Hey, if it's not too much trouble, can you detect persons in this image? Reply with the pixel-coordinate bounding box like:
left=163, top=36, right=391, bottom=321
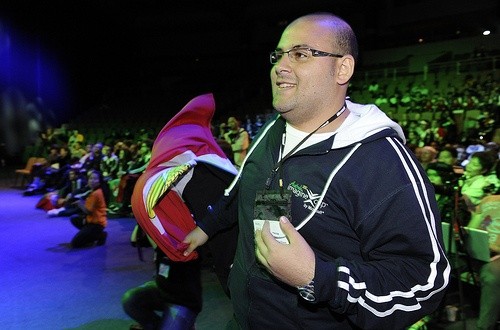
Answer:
left=23, top=79, right=500, bottom=330
left=177, top=14, right=448, bottom=330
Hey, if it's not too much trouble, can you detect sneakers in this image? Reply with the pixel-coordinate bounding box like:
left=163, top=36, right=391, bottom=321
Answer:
left=109, top=207, right=125, bottom=215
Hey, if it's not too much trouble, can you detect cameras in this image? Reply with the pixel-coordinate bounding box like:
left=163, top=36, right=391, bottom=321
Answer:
left=222, top=125, right=231, bottom=132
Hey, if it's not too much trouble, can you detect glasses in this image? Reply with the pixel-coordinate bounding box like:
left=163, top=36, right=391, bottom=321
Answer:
left=270, top=45, right=343, bottom=64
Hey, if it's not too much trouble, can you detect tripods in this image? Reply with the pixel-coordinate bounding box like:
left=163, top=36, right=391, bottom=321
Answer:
left=440, top=186, right=482, bottom=330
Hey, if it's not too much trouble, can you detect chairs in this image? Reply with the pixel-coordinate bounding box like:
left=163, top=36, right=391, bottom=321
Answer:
left=442, top=222, right=500, bottom=288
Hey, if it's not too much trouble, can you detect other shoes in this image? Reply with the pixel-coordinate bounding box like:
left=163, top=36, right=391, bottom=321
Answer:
left=16, top=170, right=30, bottom=174
left=96, top=232, right=108, bottom=246
